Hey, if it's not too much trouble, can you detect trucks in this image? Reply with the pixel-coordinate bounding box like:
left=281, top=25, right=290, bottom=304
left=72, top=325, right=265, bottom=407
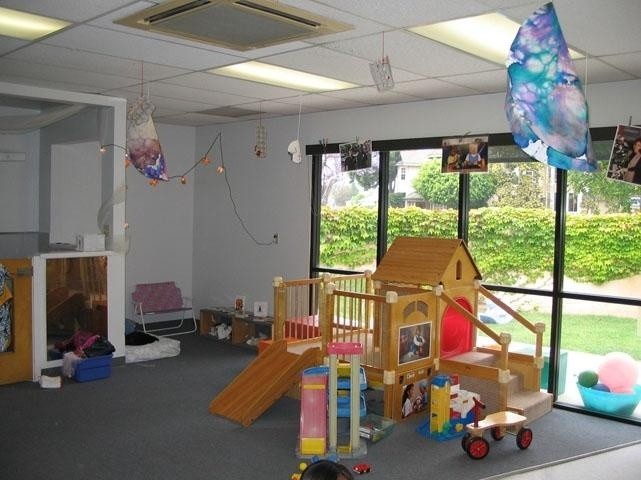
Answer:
left=630, top=197, right=641, bottom=213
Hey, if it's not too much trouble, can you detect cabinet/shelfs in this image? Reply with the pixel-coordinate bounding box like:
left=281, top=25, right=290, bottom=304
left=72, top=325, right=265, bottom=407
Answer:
left=200, top=307, right=274, bottom=352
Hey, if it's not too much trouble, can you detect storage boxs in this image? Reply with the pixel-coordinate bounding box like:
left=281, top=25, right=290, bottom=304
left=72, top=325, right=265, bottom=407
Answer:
left=60, top=351, right=113, bottom=382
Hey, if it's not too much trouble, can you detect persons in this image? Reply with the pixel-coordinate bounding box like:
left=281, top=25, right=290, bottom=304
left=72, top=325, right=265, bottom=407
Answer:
left=446, top=146, right=463, bottom=171
left=345, top=148, right=358, bottom=170
left=619, top=138, right=641, bottom=186
left=416, top=382, right=430, bottom=408
left=466, top=142, right=486, bottom=170
left=356, top=144, right=369, bottom=169
left=408, top=324, right=428, bottom=357
left=401, top=383, right=416, bottom=418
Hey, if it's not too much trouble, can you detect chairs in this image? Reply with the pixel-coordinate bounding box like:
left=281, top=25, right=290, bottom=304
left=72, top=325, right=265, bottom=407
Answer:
left=130, top=281, right=198, bottom=337
